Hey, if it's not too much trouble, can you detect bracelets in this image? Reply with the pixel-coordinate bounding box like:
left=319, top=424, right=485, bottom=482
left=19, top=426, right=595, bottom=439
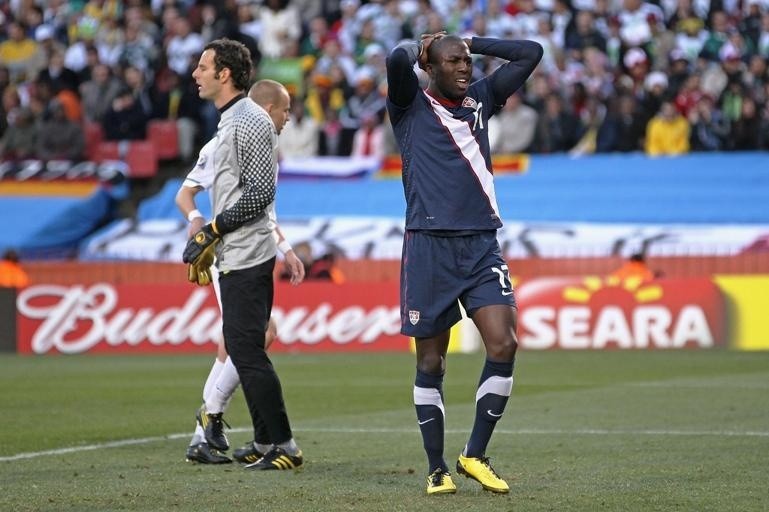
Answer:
left=416, top=41, right=425, bottom=59
left=278, top=240, right=292, bottom=255
left=186, top=209, right=203, bottom=222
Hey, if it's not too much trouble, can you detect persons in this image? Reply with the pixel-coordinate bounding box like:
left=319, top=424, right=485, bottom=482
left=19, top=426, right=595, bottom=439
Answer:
left=299, top=1, right=769, bottom=160
left=183, top=39, right=305, bottom=473
left=175, top=77, right=307, bottom=467
left=2, top=1, right=303, bottom=162
left=0, top=248, right=30, bottom=289
left=380, top=30, right=543, bottom=495
left=609, top=237, right=658, bottom=282
left=304, top=239, right=343, bottom=284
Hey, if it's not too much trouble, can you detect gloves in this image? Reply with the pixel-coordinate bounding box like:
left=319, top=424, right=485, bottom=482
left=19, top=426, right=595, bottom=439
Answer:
left=182, top=224, right=220, bottom=268
left=186, top=262, right=212, bottom=285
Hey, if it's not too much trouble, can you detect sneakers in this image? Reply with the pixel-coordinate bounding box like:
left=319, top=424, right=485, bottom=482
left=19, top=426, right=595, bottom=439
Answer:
left=233, top=440, right=263, bottom=463
left=185, top=441, right=233, bottom=465
left=195, top=403, right=232, bottom=450
left=245, top=444, right=305, bottom=470
left=456, top=453, right=509, bottom=494
left=426, top=467, right=456, bottom=495
